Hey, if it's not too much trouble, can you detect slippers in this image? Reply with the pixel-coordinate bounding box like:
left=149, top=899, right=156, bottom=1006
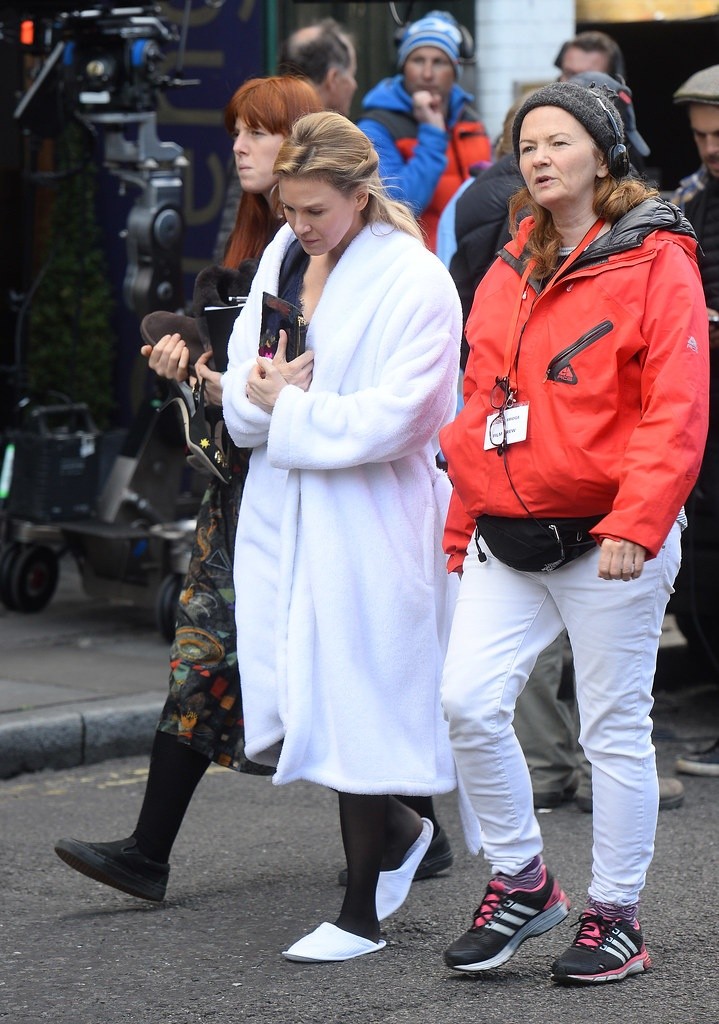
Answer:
left=374, top=817, right=434, bottom=921
left=282, top=921, right=387, bottom=961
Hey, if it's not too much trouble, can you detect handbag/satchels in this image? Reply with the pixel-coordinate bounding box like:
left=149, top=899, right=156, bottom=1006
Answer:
left=475, top=514, right=607, bottom=572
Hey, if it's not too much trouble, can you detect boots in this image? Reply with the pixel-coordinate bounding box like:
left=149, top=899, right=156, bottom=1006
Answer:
left=141, top=259, right=260, bottom=380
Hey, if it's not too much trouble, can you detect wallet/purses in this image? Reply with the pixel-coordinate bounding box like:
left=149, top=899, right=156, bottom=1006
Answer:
left=258, top=291, right=309, bottom=362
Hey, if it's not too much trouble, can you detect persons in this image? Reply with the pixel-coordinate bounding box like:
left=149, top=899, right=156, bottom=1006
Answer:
left=277, top=11, right=719, bottom=813
left=439, top=83, right=709, bottom=983
left=53, top=76, right=454, bottom=899
left=220, top=112, right=464, bottom=964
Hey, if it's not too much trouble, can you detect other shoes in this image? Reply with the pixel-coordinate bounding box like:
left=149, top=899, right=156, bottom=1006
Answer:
left=676, top=740, right=719, bottom=776
left=532, top=776, right=579, bottom=807
left=576, top=777, right=684, bottom=811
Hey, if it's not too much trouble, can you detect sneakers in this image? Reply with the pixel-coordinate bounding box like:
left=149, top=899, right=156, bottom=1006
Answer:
left=339, top=826, right=452, bottom=886
left=56, top=838, right=170, bottom=902
left=550, top=910, right=652, bottom=984
left=444, top=864, right=571, bottom=971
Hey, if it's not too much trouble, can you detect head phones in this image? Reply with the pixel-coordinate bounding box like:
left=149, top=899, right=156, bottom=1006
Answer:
left=590, top=89, right=630, bottom=179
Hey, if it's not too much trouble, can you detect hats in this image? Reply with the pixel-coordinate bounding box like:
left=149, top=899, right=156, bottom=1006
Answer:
left=674, top=64, right=719, bottom=104
left=569, top=71, right=651, bottom=156
left=511, top=81, right=630, bottom=179
left=397, top=10, right=463, bottom=83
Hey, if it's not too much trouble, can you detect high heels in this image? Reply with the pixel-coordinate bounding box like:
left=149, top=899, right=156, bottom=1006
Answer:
left=156, top=377, right=233, bottom=485
